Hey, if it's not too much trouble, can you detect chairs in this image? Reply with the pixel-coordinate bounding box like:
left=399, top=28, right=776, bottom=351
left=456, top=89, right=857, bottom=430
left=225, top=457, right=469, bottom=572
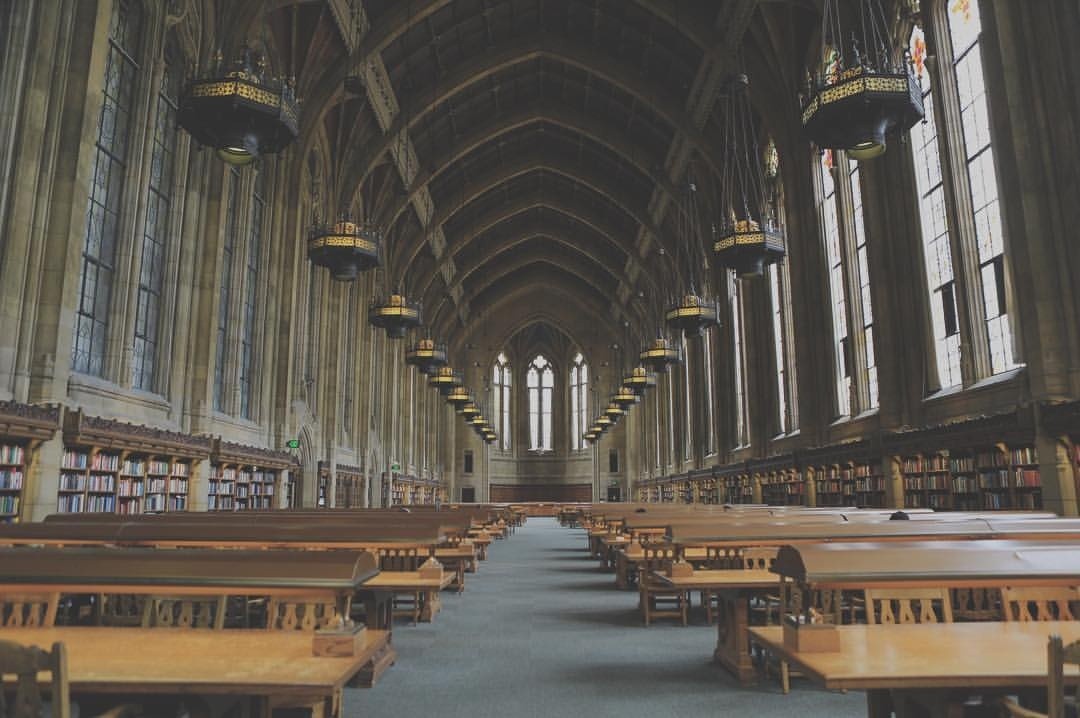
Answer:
left=1, top=508, right=1080, bottom=718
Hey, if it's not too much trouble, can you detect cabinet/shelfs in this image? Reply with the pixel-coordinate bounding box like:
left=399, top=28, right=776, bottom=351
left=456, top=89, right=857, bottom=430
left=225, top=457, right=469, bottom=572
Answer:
left=2, top=397, right=1079, bottom=520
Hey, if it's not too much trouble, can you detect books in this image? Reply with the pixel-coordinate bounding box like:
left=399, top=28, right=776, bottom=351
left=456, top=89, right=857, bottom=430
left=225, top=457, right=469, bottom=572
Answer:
left=318, top=473, right=372, bottom=509
left=639, top=479, right=718, bottom=506
left=393, top=484, right=450, bottom=506
left=722, top=478, right=752, bottom=504
left=903, top=444, right=1042, bottom=511
left=55, top=443, right=190, bottom=514
left=0, top=443, right=27, bottom=524
left=813, top=461, right=886, bottom=508
left=761, top=470, right=804, bottom=505
left=208, top=464, right=276, bottom=511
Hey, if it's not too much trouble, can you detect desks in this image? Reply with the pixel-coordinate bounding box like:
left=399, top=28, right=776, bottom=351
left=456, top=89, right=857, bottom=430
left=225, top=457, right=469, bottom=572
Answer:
left=362, top=570, right=457, bottom=622
left=746, top=621, right=1080, bottom=718
left=469, top=523, right=509, bottom=537
left=448, top=536, right=490, bottom=560
left=599, top=536, right=654, bottom=572
left=613, top=550, right=741, bottom=589
left=466, top=529, right=503, bottom=544
left=588, top=530, right=620, bottom=559
left=0, top=623, right=389, bottom=718
left=379, top=547, right=478, bottom=592
left=639, top=565, right=791, bottom=625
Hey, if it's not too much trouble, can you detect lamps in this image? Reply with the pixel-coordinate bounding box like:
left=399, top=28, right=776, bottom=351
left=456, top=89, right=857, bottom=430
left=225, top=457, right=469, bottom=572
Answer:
left=176, top=1, right=923, bottom=445
